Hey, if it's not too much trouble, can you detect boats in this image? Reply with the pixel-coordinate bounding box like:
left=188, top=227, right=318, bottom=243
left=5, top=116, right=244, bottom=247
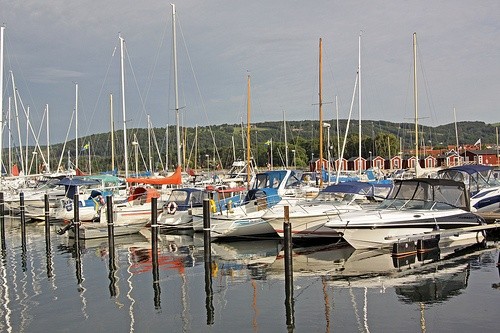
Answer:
left=323, top=176, right=488, bottom=250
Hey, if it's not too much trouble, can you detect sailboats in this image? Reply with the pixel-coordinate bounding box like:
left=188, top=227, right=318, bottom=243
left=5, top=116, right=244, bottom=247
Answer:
left=0, top=2, right=500, bottom=240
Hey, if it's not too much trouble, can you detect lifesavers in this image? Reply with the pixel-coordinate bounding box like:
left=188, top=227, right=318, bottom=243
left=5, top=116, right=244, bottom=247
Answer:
left=167, top=201, right=177, bottom=214
left=210, top=200, right=217, bottom=213
